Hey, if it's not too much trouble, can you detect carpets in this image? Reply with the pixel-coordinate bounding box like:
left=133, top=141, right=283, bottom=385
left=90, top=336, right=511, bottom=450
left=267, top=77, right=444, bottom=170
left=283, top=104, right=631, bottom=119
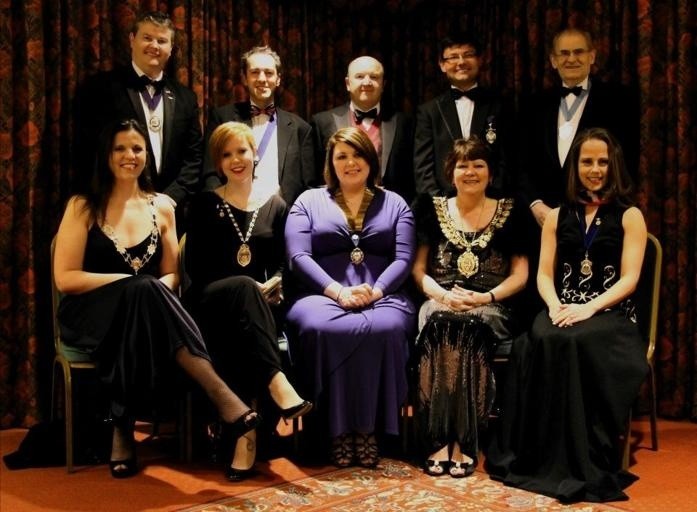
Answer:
left=174, top=457, right=634, bottom=512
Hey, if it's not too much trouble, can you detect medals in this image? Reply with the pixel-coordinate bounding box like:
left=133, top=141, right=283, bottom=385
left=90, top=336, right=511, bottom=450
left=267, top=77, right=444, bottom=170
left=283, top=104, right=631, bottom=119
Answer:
left=579, top=260, right=593, bottom=276
left=457, top=249, right=479, bottom=279
left=351, top=247, right=363, bottom=266
left=237, top=244, right=252, bottom=266
left=149, top=113, right=161, bottom=132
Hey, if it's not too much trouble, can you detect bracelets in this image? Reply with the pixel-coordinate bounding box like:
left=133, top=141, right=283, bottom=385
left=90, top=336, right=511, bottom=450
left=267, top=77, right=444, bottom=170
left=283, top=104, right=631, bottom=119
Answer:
left=336, top=287, right=344, bottom=302
left=488, top=289, right=495, bottom=303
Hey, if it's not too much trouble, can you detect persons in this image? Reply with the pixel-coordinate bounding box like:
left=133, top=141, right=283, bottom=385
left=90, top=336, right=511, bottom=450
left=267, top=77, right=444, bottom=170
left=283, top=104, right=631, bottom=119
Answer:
left=202, top=45, right=316, bottom=219
left=70, top=13, right=203, bottom=205
left=412, top=133, right=531, bottom=477
left=284, top=127, right=416, bottom=469
left=185, top=121, right=314, bottom=479
left=54, top=118, right=265, bottom=479
left=517, top=27, right=641, bottom=230
left=311, top=56, right=417, bottom=206
left=483, top=126, right=650, bottom=503
left=413, top=34, right=518, bottom=199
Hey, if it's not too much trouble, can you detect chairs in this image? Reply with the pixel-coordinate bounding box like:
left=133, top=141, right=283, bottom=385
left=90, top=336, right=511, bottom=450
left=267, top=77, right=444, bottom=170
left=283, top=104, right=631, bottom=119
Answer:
left=488, top=325, right=514, bottom=365
left=49, top=231, right=192, bottom=475
left=622, top=230, right=664, bottom=471
left=282, top=330, right=407, bottom=458
left=177, top=231, right=190, bottom=297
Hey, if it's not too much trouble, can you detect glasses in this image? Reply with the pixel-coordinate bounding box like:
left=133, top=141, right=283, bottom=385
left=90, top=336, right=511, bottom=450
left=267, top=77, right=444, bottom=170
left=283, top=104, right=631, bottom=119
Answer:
left=443, top=51, right=474, bottom=60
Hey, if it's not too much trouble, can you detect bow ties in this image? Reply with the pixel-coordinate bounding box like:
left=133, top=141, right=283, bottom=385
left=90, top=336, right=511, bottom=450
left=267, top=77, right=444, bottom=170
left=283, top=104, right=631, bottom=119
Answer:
left=250, top=106, right=276, bottom=117
left=450, top=87, right=478, bottom=100
left=138, top=75, right=164, bottom=90
left=353, top=109, right=377, bottom=121
left=560, top=87, right=582, bottom=97
